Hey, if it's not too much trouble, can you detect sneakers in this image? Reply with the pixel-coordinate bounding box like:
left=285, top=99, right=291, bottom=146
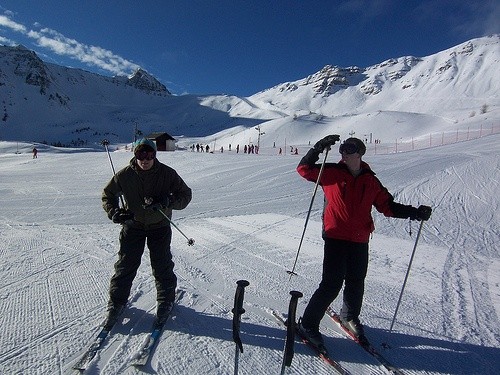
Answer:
left=158, top=195, right=172, bottom=209
left=340, top=309, right=364, bottom=338
left=107, top=304, right=123, bottom=320
left=296, top=323, right=324, bottom=349
left=157, top=302, right=173, bottom=318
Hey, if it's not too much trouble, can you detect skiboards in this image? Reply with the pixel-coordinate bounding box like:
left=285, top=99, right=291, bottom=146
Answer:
left=291, top=154, right=299, bottom=155
left=271, top=306, right=403, bottom=375
left=73, top=289, right=183, bottom=370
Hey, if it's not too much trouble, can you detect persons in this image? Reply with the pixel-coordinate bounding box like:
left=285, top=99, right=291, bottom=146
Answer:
left=244, top=144, right=259, bottom=155
left=229, top=144, right=231, bottom=151
left=298, top=134, right=431, bottom=351
left=374, top=139, right=381, bottom=144
left=190, top=143, right=210, bottom=153
left=279, top=148, right=282, bottom=156
left=125, top=144, right=128, bottom=150
left=220, top=147, right=223, bottom=153
left=33, top=147, right=37, bottom=159
left=290, top=145, right=293, bottom=152
left=308, top=141, right=310, bottom=145
left=295, top=148, right=298, bottom=155
left=365, top=139, right=367, bottom=143
left=102, top=138, right=191, bottom=333
left=236, top=145, right=239, bottom=154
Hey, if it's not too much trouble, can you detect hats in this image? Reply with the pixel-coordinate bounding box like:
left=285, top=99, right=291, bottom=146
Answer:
left=133, top=137, right=156, bottom=155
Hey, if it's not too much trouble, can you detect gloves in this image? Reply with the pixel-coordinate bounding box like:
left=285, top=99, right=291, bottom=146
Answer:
left=314, top=135, right=340, bottom=153
left=112, top=208, right=134, bottom=223
left=410, top=205, right=432, bottom=221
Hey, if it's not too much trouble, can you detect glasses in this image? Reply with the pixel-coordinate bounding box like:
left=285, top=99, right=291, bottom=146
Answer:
left=135, top=150, right=155, bottom=161
left=339, top=144, right=358, bottom=154
